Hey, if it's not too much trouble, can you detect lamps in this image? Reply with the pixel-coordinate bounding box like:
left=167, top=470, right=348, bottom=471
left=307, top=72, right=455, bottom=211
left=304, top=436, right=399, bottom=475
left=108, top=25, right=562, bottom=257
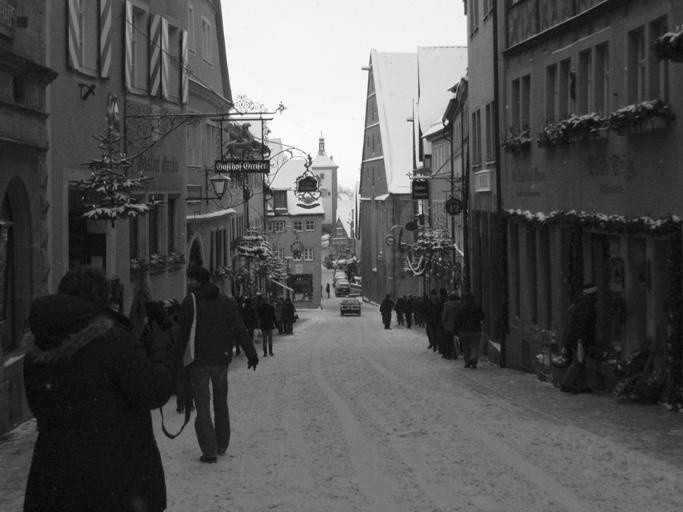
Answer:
left=185, top=167, right=231, bottom=203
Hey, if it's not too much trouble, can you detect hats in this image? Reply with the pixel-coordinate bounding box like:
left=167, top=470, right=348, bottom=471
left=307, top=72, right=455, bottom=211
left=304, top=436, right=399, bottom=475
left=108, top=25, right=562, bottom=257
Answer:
left=187, top=265, right=210, bottom=283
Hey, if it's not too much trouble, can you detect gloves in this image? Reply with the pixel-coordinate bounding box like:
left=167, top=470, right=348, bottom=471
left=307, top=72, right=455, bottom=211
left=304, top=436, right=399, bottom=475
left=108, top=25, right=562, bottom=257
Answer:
left=247, top=353, right=259, bottom=371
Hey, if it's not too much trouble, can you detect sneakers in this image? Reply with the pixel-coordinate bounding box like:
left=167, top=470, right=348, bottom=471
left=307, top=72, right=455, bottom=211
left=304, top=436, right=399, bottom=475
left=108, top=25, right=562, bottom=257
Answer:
left=200, top=454, right=217, bottom=463
left=428, top=344, right=478, bottom=369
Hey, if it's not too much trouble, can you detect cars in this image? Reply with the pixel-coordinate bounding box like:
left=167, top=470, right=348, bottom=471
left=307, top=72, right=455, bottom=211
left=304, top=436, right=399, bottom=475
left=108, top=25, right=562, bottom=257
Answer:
left=332, top=267, right=362, bottom=318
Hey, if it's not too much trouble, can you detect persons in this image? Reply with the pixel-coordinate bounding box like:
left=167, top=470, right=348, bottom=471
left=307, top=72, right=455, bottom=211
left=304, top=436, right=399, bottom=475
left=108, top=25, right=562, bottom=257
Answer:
left=378, top=287, right=486, bottom=371
left=171, top=264, right=260, bottom=464
left=22, top=271, right=173, bottom=512
left=564, top=274, right=597, bottom=361
left=231, top=291, right=297, bottom=359
left=325, top=282, right=331, bottom=299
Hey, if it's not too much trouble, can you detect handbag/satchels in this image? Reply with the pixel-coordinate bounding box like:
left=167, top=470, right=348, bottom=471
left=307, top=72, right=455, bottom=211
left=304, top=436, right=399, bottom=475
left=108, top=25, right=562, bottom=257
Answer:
left=182, top=327, right=196, bottom=368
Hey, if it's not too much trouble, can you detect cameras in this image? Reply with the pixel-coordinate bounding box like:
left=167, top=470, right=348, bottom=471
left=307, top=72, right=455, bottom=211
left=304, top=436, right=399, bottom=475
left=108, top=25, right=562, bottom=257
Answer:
left=145, top=298, right=181, bottom=326
